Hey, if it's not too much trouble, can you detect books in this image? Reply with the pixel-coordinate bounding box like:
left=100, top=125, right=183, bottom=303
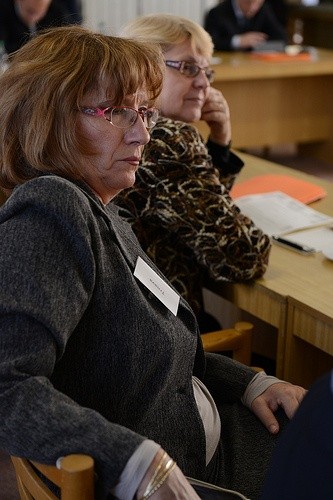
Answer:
left=227, top=173, right=333, bottom=259
left=246, top=37, right=312, bottom=65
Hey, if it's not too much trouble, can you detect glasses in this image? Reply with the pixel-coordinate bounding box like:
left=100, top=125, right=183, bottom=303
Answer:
left=55, top=104, right=161, bottom=129
left=164, top=59, right=215, bottom=82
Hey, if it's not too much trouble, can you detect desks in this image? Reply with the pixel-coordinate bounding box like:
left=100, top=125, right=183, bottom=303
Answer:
left=283, top=272, right=333, bottom=389
left=188, top=45, right=332, bottom=172
left=200, top=147, right=333, bottom=381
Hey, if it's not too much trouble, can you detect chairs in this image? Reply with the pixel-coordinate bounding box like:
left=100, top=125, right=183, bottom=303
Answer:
left=9, top=453, right=95, bottom=500
left=194, top=320, right=254, bottom=368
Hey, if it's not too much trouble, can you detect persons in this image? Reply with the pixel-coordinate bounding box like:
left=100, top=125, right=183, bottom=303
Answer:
left=0, top=25, right=308, bottom=500
left=0, top=0, right=81, bottom=65
left=205, top=0, right=289, bottom=53
left=117, top=13, right=275, bottom=361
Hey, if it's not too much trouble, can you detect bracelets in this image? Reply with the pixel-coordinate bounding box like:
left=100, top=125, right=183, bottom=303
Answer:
left=139, top=451, right=177, bottom=500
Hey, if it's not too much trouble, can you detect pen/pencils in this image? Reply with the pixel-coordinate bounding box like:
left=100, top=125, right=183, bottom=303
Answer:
left=272, top=234, right=310, bottom=253
